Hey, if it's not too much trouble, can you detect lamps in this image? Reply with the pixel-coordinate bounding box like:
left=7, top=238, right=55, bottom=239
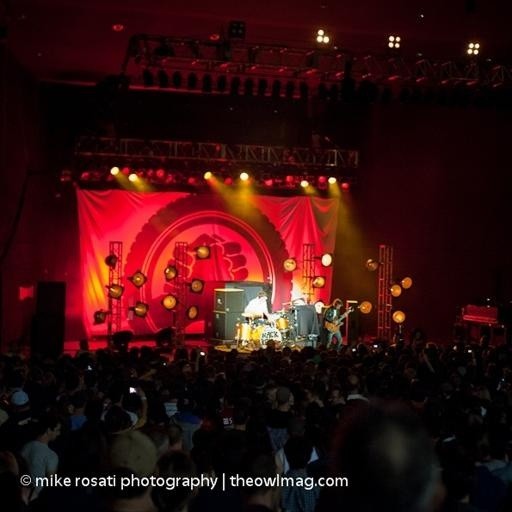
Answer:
left=94, top=245, right=415, bottom=324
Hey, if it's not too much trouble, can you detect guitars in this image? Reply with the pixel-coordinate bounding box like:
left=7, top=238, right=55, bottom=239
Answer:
left=324, top=306, right=354, bottom=334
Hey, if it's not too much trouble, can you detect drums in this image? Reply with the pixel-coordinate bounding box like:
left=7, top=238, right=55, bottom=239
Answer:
left=252, top=325, right=282, bottom=350
left=254, top=318, right=271, bottom=327
left=275, top=316, right=290, bottom=333
left=234, top=324, right=241, bottom=341
left=240, top=323, right=252, bottom=341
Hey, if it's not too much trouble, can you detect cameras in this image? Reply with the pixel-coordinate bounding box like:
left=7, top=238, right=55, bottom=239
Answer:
left=372, top=344, right=379, bottom=349
left=467, top=349, right=473, bottom=354
left=199, top=351, right=206, bottom=356
left=351, top=347, right=357, bottom=353
left=129, top=386, right=137, bottom=394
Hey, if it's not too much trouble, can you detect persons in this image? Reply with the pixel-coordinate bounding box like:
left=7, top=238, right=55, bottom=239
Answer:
left=2, top=322, right=511, bottom=512
left=240, top=289, right=270, bottom=323
left=320, top=297, right=348, bottom=351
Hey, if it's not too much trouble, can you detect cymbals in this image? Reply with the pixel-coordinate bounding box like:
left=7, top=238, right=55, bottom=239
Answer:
left=274, top=310, right=289, bottom=313
left=241, top=312, right=262, bottom=319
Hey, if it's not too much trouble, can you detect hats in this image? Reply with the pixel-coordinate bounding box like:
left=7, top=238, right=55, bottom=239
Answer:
left=106, top=429, right=160, bottom=480
left=10, top=391, right=30, bottom=406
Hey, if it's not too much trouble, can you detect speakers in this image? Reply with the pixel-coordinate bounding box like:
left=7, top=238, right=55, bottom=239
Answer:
left=112, top=330, right=135, bottom=352
left=213, top=311, right=241, bottom=340
left=297, top=305, right=319, bottom=336
left=213, top=288, right=245, bottom=314
left=32, top=280, right=66, bottom=359
left=155, top=326, right=177, bottom=346
